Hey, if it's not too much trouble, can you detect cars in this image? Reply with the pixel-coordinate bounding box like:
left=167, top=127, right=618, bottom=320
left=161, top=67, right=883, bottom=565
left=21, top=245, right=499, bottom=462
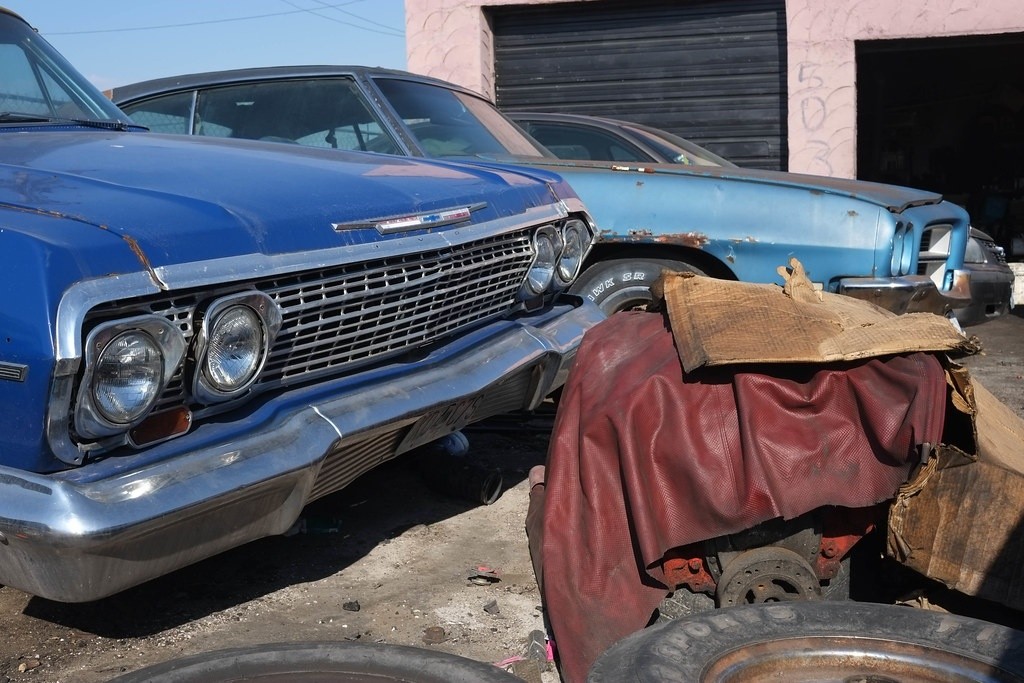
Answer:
left=953, top=226, right=1015, bottom=328
left=49, top=64, right=969, bottom=346
left=351, top=111, right=744, bottom=171
left=0, top=7, right=611, bottom=605
